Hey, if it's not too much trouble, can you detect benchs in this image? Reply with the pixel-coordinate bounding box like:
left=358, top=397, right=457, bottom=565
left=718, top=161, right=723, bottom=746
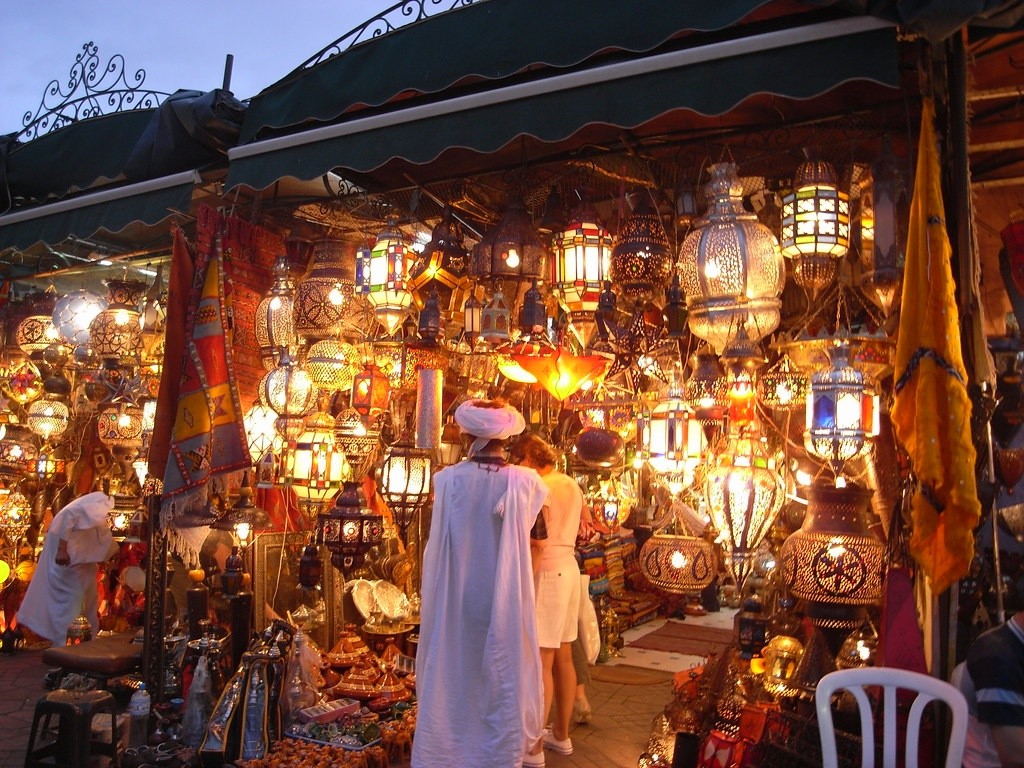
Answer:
left=39, top=628, right=144, bottom=741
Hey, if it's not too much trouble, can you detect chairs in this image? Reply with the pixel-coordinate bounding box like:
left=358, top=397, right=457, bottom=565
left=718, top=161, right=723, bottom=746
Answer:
left=816, top=667, right=969, bottom=768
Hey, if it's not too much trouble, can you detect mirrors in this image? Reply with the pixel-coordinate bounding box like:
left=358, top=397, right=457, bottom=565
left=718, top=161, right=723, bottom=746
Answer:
left=145, top=494, right=252, bottom=743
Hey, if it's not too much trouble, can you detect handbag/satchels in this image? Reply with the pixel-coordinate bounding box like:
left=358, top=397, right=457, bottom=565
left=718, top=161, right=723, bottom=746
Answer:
left=577, top=574, right=600, bottom=665
left=868, top=566, right=930, bottom=705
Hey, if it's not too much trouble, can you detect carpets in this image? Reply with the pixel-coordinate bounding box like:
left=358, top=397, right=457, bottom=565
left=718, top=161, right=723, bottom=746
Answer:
left=623, top=619, right=732, bottom=657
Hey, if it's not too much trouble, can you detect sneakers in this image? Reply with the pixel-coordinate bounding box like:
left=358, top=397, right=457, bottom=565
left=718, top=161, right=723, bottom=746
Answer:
left=524, top=751, right=546, bottom=768
left=543, top=725, right=573, bottom=756
left=574, top=699, right=592, bottom=723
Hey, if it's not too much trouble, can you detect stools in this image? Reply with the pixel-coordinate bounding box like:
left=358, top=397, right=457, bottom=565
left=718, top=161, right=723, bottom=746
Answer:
left=24, top=690, right=118, bottom=768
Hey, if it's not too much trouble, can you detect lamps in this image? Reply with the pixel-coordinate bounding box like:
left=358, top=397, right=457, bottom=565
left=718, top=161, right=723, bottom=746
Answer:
left=0, top=147, right=891, bottom=698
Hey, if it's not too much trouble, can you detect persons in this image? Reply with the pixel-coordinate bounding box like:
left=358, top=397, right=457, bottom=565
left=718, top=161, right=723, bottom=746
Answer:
left=975, top=373, right=1023, bottom=560
left=407, top=397, right=594, bottom=768
left=958, top=572, right=1024, bottom=768
left=16, top=490, right=114, bottom=649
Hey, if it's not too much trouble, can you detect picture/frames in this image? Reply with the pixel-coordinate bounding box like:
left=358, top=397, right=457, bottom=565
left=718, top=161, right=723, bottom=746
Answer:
left=253, top=531, right=343, bottom=652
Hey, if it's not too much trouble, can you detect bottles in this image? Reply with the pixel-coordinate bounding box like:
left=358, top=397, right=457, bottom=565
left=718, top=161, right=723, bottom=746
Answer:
left=767, top=598, right=800, bottom=641
left=740, top=601, right=767, bottom=659
left=128, top=683, right=150, bottom=747
